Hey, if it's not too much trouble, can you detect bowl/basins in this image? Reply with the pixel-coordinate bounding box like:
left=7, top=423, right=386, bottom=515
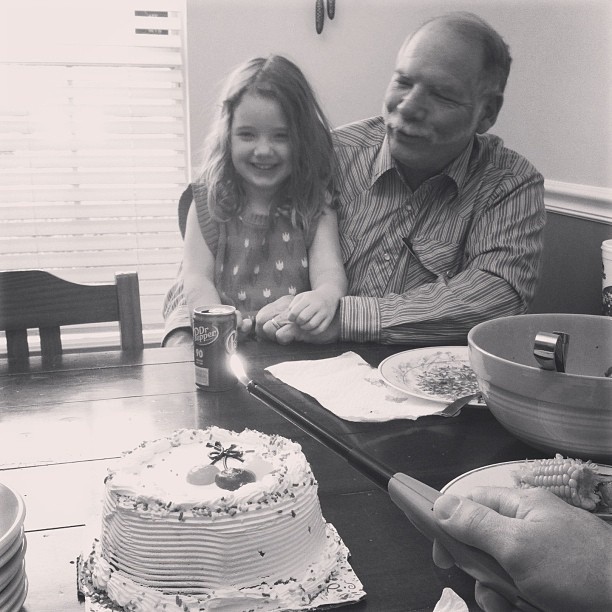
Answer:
left=467, top=312, right=612, bottom=462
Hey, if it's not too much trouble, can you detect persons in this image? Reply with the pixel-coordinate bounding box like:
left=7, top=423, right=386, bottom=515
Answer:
left=432, top=485, right=612, bottom=611
left=161, top=11, right=547, bottom=348
left=178, top=55, right=349, bottom=344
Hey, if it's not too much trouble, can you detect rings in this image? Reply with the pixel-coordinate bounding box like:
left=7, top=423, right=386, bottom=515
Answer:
left=271, top=318, right=281, bottom=329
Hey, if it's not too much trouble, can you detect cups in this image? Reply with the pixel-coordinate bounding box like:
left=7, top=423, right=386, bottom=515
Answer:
left=600, top=238, right=612, bottom=317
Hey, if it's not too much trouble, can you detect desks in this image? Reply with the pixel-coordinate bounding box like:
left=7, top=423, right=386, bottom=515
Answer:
left=1, top=345, right=547, bottom=611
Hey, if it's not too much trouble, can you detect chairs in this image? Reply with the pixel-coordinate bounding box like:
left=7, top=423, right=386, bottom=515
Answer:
left=178, top=183, right=193, bottom=239
left=0, top=270, right=143, bottom=355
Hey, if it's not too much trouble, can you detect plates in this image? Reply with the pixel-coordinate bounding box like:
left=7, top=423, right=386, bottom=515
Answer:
left=0, top=574, right=25, bottom=612
left=438, top=458, right=612, bottom=525
left=0, top=558, right=26, bottom=607
left=0, top=526, right=20, bottom=557
left=0, top=568, right=21, bottom=593
left=377, top=343, right=487, bottom=408
left=0, top=533, right=28, bottom=586
left=0, top=480, right=26, bottom=550
left=10, top=583, right=29, bottom=612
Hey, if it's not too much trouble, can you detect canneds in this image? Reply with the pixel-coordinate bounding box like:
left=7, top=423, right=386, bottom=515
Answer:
left=192, top=304, right=239, bottom=390
